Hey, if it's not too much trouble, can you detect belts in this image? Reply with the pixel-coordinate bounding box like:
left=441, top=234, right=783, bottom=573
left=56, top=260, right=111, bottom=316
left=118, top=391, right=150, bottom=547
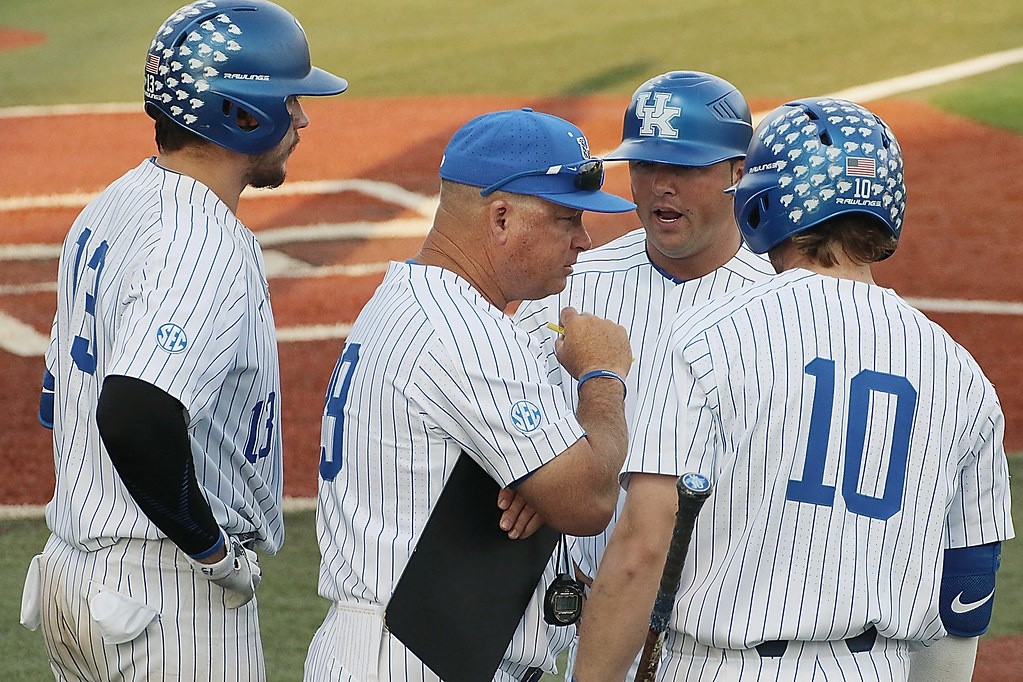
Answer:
left=520, top=666, right=544, bottom=682
left=755, top=626, right=877, bottom=658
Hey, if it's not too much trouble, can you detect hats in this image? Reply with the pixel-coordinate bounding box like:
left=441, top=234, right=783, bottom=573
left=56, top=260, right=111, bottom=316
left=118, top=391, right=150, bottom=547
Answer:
left=437, top=106, right=637, bottom=214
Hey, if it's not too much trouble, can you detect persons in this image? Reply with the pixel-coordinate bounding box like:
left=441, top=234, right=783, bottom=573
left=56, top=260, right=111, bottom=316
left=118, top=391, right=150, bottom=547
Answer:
left=513, top=72, right=777, bottom=682
left=573, top=96, right=1016, bottom=682
left=20, top=0, right=347, bottom=682
left=304, top=108, right=633, bottom=682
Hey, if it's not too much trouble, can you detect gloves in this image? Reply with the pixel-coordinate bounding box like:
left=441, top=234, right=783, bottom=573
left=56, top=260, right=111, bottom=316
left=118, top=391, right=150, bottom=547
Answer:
left=181, top=526, right=261, bottom=609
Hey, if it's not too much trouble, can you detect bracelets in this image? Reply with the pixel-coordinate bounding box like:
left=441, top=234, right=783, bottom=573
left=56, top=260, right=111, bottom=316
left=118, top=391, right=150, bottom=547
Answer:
left=577, top=370, right=627, bottom=400
left=190, top=531, right=223, bottom=560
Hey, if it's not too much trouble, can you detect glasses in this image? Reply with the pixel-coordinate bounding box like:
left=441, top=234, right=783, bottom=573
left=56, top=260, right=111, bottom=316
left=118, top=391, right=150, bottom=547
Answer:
left=479, top=156, right=605, bottom=198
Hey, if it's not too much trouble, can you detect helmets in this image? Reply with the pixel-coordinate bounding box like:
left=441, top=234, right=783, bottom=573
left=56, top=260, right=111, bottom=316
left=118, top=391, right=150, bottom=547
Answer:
left=722, top=97, right=907, bottom=265
left=603, top=70, right=753, bottom=166
left=144, top=0, right=348, bottom=155
